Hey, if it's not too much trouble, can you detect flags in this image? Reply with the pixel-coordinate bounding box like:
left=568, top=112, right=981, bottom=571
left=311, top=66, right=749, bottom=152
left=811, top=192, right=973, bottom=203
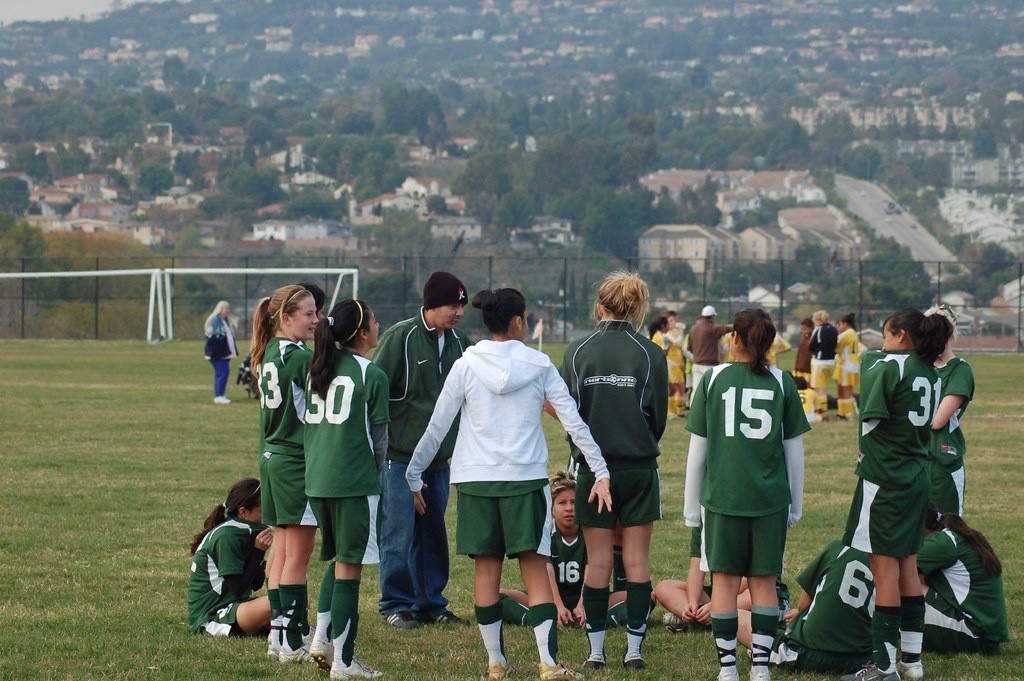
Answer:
left=531, top=320, right=543, bottom=340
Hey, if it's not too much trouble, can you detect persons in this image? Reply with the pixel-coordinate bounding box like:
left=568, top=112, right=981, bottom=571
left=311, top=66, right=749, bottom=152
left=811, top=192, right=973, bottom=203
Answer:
left=913, top=501, right=1013, bottom=657
left=840, top=305, right=953, bottom=681
left=922, top=306, right=975, bottom=520
left=683, top=307, right=811, bottom=680
left=687, top=304, right=734, bottom=409
left=649, top=309, right=694, bottom=419
left=496, top=468, right=656, bottom=632
left=832, top=311, right=868, bottom=423
left=783, top=536, right=878, bottom=671
left=785, top=371, right=829, bottom=424
left=651, top=502, right=786, bottom=634
left=203, top=300, right=238, bottom=405
left=763, top=327, right=791, bottom=369
left=809, top=309, right=838, bottom=420
left=369, top=273, right=476, bottom=631
left=300, top=296, right=387, bottom=679
left=405, top=286, right=612, bottom=681
left=187, top=478, right=271, bottom=638
left=794, top=317, right=815, bottom=388
left=248, top=278, right=325, bottom=664
left=544, top=269, right=668, bottom=669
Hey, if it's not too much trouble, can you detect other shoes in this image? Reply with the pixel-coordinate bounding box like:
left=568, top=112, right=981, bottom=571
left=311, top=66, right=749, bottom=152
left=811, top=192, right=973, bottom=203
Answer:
left=718, top=671, right=740, bottom=681
left=662, top=612, right=695, bottom=633
left=584, top=654, right=605, bottom=670
left=215, top=396, right=231, bottom=404
left=623, top=651, right=645, bottom=668
left=749, top=670, right=771, bottom=681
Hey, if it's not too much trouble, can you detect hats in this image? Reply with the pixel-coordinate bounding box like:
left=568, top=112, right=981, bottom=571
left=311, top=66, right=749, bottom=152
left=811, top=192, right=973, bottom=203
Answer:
left=701, top=305, right=718, bottom=316
left=423, top=271, right=467, bottom=309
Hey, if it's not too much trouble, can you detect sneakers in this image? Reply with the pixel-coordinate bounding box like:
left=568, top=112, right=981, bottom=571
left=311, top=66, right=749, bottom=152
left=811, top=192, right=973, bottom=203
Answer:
left=897, top=658, right=923, bottom=681
left=386, top=611, right=419, bottom=629
left=487, top=662, right=519, bottom=678
left=279, top=643, right=312, bottom=664
left=539, top=662, right=583, bottom=681
left=329, top=658, right=383, bottom=679
left=840, top=660, right=902, bottom=681
left=266, top=646, right=280, bottom=660
left=424, top=610, right=470, bottom=626
left=309, top=637, right=335, bottom=671
